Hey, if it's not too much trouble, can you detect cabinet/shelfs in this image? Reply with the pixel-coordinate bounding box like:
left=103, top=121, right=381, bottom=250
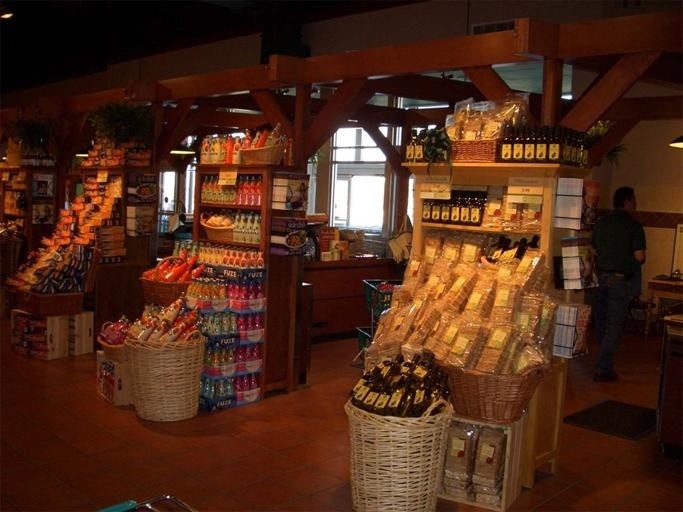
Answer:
left=0, top=172, right=158, bottom=345
left=410, top=173, right=567, bottom=490
left=190, top=168, right=308, bottom=399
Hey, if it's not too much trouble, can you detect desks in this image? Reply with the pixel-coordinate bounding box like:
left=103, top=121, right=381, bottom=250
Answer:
left=641, top=272, right=682, bottom=441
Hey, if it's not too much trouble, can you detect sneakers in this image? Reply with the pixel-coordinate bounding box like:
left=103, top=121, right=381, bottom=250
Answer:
left=592, top=368, right=618, bottom=382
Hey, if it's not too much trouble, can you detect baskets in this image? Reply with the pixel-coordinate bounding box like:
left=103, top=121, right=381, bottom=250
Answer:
left=361, top=278, right=402, bottom=318
left=98, top=322, right=129, bottom=362
left=449, top=139, right=501, bottom=163
left=200, top=212, right=234, bottom=243
left=139, top=256, right=192, bottom=307
left=7, top=148, right=22, bottom=165
left=16, top=286, right=85, bottom=316
left=437, top=360, right=544, bottom=424
left=343, top=398, right=454, bottom=511
left=2, top=238, right=23, bottom=276
left=358, top=327, right=379, bottom=360
left=124, top=330, right=204, bottom=422
left=240, top=143, right=284, bottom=166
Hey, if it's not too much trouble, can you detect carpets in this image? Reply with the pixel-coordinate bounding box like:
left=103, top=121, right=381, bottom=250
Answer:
left=562, top=398, right=656, bottom=439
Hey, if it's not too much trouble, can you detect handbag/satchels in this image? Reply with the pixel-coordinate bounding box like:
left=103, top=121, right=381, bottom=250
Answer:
left=388, top=215, right=413, bottom=262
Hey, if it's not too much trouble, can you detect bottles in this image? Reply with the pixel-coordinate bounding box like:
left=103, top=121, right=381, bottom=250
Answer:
left=406, top=126, right=600, bottom=263
left=95, top=122, right=294, bottom=400
left=349, top=351, right=451, bottom=416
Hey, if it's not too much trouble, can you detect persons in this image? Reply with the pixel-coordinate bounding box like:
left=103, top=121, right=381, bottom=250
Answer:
left=590, top=186, right=646, bottom=383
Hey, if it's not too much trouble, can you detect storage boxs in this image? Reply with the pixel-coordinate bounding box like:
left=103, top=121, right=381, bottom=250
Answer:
left=93, top=351, right=133, bottom=407
left=9, top=308, right=95, bottom=363
